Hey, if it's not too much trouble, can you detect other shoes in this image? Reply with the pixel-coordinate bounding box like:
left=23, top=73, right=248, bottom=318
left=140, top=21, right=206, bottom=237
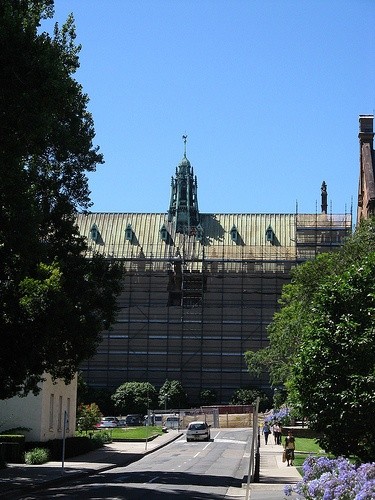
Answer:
left=265, top=442, right=268, bottom=445
left=275, top=441, right=282, bottom=445
left=287, top=463, right=293, bottom=466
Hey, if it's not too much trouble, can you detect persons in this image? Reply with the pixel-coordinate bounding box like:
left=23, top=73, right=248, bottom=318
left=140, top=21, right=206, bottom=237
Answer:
left=272, top=422, right=282, bottom=445
left=284, top=431, right=295, bottom=467
left=262, top=421, right=271, bottom=445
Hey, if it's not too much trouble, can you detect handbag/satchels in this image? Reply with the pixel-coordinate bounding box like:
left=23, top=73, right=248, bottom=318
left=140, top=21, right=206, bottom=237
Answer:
left=268, top=428, right=271, bottom=434
left=283, top=451, right=286, bottom=463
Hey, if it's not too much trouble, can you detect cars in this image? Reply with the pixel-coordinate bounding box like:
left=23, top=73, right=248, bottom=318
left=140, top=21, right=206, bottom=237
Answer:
left=76, top=413, right=179, bottom=429
left=185, top=421, right=212, bottom=442
